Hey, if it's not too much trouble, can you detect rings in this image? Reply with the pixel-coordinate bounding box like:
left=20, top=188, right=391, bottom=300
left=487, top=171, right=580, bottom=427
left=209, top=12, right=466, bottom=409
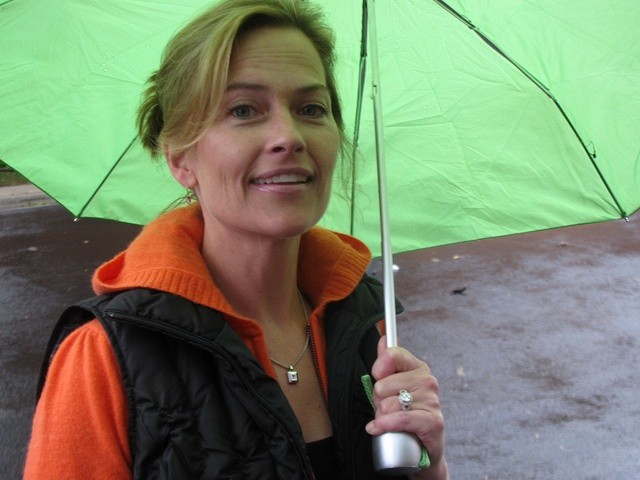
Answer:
left=400, top=388, right=413, bottom=412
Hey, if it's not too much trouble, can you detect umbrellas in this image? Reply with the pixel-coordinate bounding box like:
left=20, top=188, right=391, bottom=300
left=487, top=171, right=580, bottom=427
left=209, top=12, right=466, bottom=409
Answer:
left=0, top=0, right=640, bottom=471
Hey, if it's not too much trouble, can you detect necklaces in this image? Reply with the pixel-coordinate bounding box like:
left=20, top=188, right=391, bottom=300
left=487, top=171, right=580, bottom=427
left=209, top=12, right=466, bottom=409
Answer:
left=267, top=347, right=310, bottom=384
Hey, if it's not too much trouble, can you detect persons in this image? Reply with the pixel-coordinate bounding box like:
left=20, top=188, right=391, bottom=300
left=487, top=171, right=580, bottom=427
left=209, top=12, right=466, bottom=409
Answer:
left=23, top=0, right=448, bottom=480
